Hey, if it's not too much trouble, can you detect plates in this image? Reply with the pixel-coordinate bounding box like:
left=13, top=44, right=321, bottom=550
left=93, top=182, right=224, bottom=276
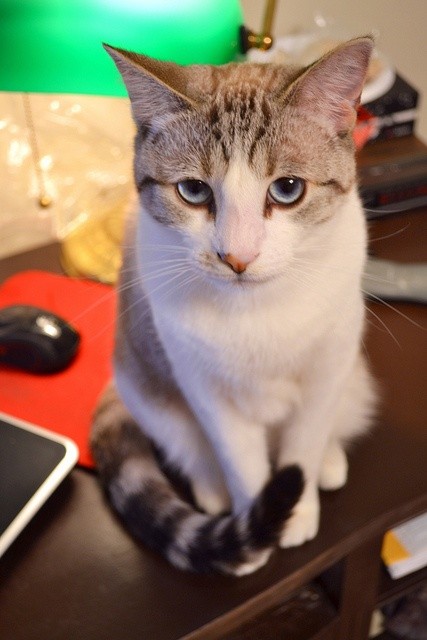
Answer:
left=246, top=32, right=397, bottom=106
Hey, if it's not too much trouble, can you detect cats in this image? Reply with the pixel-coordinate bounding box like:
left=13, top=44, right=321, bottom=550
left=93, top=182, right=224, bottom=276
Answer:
left=88, top=34, right=427, bottom=579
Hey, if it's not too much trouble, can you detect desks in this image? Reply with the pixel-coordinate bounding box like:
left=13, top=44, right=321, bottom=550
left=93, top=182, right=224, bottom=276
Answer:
left=0, top=208, right=427, bottom=640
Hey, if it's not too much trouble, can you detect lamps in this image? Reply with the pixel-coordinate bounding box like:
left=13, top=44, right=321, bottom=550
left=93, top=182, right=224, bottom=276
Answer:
left=0, top=2, right=282, bottom=104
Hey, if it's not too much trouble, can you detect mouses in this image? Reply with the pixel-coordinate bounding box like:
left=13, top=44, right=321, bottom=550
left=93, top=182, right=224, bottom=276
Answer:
left=0, top=305, right=80, bottom=374
